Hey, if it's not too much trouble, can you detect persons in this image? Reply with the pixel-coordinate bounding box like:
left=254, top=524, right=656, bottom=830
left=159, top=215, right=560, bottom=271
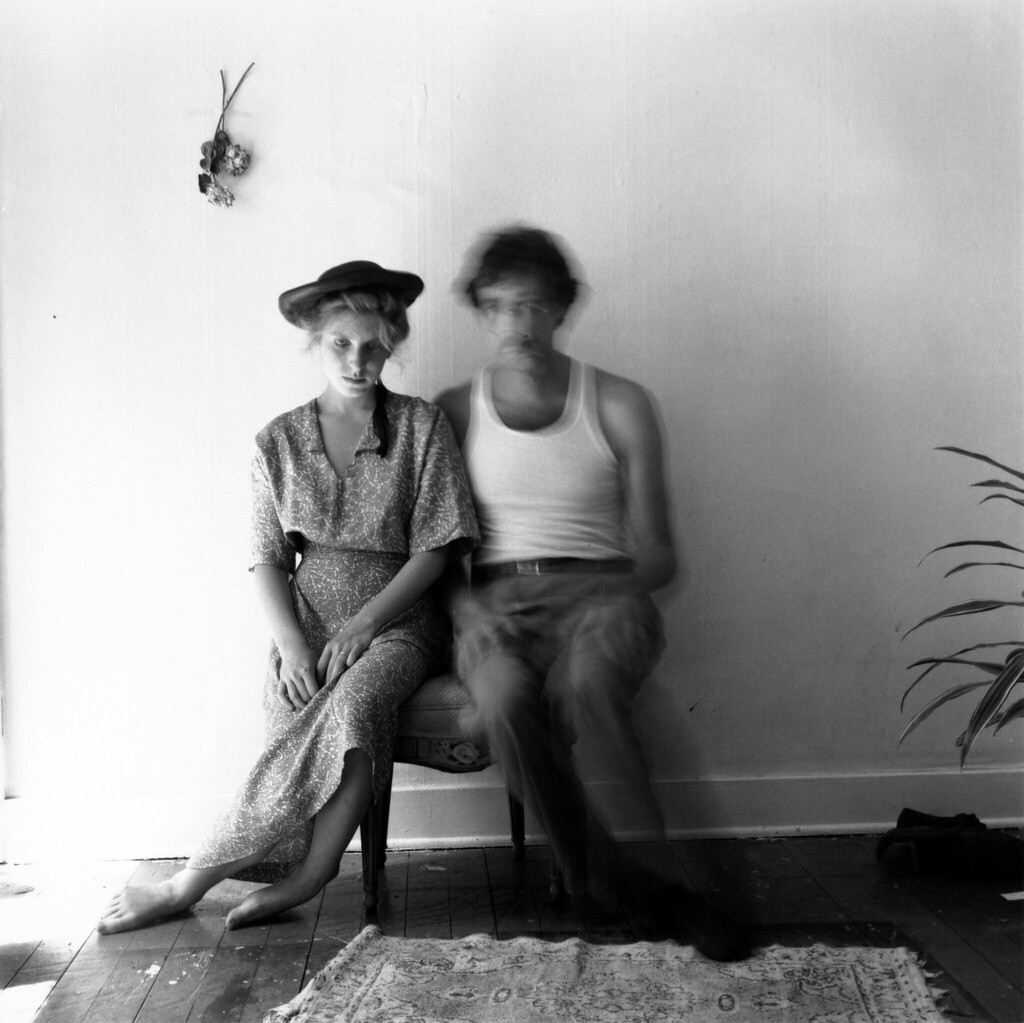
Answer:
left=422, top=224, right=761, bottom=967
left=88, top=261, right=477, bottom=934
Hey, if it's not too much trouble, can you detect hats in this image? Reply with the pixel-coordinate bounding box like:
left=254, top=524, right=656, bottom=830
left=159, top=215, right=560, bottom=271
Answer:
left=279, top=261, right=424, bottom=330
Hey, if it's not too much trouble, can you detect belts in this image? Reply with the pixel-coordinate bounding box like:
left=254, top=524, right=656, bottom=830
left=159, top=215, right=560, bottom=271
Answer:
left=460, top=555, right=633, bottom=587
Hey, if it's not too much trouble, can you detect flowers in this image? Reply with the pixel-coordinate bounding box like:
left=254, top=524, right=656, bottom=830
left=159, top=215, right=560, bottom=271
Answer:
left=197, top=62, right=256, bottom=207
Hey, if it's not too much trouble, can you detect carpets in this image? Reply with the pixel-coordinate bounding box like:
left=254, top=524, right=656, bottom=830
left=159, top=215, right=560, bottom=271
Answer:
left=260, top=926, right=956, bottom=1023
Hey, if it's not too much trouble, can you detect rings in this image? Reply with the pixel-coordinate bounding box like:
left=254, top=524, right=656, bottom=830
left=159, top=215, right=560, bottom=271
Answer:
left=340, top=652, right=349, bottom=657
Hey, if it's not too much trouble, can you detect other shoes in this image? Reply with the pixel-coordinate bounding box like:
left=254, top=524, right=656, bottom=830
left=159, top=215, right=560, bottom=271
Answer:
left=876, top=809, right=1024, bottom=879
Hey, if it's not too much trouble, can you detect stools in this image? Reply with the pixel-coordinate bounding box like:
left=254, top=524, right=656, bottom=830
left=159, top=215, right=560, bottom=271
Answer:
left=349, top=667, right=566, bottom=932
